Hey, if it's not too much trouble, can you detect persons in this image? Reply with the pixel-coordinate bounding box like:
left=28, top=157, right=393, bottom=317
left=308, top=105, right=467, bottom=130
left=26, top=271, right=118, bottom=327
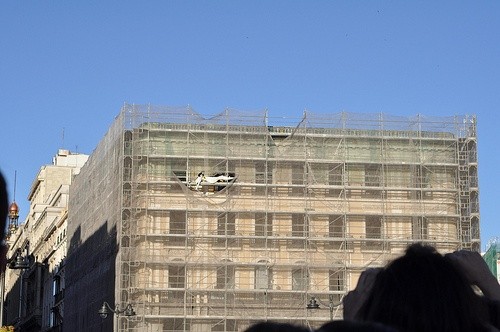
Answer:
left=242, top=241, right=500, bottom=332
left=0, top=171, right=11, bottom=282
